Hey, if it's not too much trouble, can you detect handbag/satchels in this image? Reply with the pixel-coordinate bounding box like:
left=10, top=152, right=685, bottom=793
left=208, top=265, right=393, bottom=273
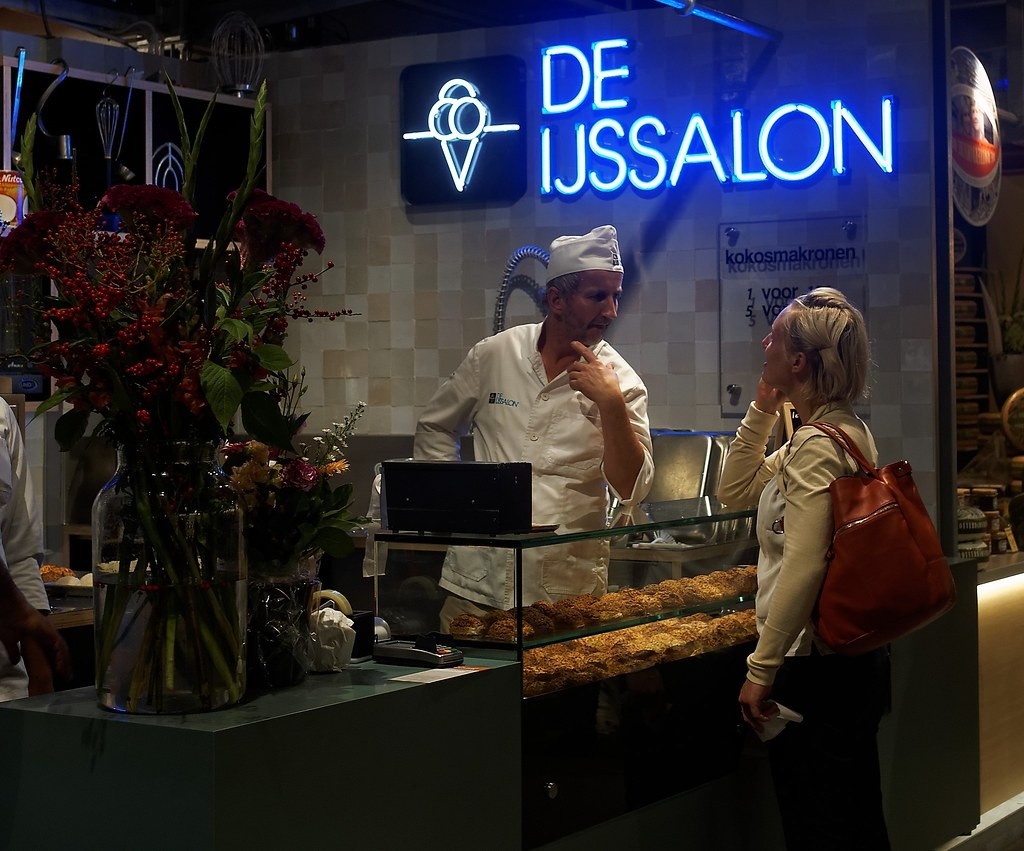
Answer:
left=779, top=421, right=957, bottom=654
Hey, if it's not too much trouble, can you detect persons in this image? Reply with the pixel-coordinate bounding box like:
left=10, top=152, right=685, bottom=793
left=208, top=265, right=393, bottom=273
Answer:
left=412, top=224, right=656, bottom=635
left=716, top=287, right=892, bottom=851
left=0, top=395, right=69, bottom=702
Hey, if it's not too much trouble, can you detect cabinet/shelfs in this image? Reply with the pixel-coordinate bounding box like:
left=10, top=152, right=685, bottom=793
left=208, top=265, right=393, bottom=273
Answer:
left=0, top=498, right=758, bottom=851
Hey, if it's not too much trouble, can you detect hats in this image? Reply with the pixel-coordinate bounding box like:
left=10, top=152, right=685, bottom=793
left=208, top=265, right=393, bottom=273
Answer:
left=546, top=224, right=622, bottom=282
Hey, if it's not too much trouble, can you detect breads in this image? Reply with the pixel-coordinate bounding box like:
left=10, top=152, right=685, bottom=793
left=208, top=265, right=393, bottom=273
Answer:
left=447, top=563, right=758, bottom=695
left=39, top=564, right=93, bottom=587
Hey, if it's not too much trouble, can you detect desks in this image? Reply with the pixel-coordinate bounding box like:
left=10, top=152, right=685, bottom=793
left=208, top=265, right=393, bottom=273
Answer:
left=371, top=535, right=758, bottom=620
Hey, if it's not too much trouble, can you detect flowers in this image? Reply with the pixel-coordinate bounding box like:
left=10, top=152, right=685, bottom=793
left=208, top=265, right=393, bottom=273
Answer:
left=0, top=178, right=352, bottom=446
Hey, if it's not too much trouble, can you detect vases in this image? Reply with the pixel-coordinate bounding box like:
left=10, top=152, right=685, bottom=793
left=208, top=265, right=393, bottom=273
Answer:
left=90, top=442, right=244, bottom=716
